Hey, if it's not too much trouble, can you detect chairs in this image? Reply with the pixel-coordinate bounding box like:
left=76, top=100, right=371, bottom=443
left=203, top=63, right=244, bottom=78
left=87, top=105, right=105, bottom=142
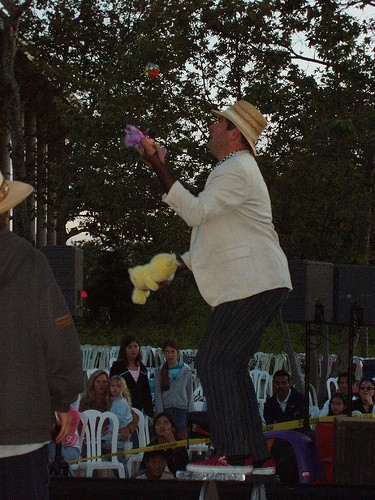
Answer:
left=80, top=409, right=126, bottom=479
left=70, top=343, right=339, bottom=410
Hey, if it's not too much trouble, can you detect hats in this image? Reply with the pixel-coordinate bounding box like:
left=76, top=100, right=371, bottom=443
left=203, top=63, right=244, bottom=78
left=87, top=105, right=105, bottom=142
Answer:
left=211, top=99, right=267, bottom=157
left=0, top=171, right=34, bottom=214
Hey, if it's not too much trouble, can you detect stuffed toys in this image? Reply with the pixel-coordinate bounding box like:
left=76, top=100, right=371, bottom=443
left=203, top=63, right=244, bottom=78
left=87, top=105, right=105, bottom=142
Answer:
left=129, top=253, right=179, bottom=304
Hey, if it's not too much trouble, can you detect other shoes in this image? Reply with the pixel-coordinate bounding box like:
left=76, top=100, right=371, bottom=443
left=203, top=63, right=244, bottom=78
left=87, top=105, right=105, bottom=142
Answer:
left=186, top=453, right=253, bottom=474
left=252, top=457, right=276, bottom=475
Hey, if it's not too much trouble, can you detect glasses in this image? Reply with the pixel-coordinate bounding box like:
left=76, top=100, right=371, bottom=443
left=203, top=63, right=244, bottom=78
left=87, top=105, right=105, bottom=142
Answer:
left=360, top=386, right=375, bottom=390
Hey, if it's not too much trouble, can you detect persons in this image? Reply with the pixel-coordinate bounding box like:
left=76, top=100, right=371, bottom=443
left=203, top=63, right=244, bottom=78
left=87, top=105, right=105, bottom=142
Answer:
left=134, top=100, right=292, bottom=475
left=47, top=333, right=375, bottom=486
left=0, top=171, right=84, bottom=500
